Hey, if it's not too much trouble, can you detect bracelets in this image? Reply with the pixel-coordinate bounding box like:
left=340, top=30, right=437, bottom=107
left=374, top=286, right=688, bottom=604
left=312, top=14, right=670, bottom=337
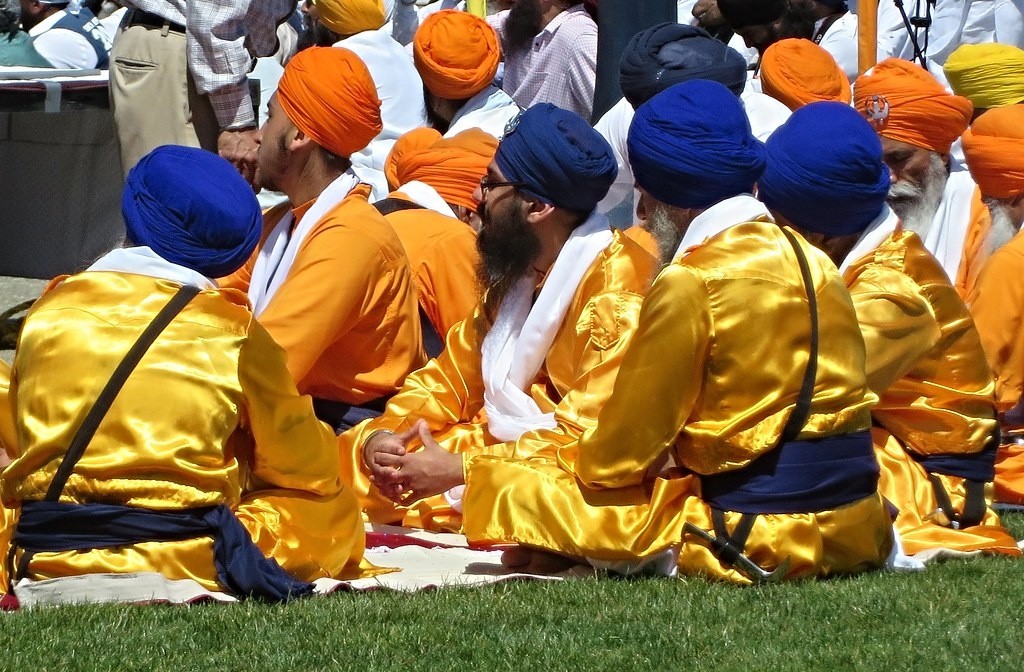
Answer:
left=362, top=429, right=401, bottom=472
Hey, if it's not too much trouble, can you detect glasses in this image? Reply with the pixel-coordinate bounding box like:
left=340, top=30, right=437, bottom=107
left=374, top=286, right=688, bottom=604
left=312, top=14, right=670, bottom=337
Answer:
left=480, top=174, right=515, bottom=201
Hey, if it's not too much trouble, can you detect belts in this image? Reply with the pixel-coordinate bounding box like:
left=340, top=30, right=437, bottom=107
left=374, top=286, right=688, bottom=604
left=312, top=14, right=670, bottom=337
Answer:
left=130, top=9, right=186, bottom=35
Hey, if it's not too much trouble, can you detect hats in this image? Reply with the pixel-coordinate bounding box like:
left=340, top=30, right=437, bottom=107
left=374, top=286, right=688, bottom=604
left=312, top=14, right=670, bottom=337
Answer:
left=121, top=145, right=264, bottom=280
left=412, top=10, right=500, bottom=100
left=852, top=60, right=974, bottom=154
left=626, top=79, right=769, bottom=210
left=619, top=21, right=747, bottom=110
left=494, top=101, right=618, bottom=217
left=755, top=101, right=891, bottom=236
left=760, top=37, right=851, bottom=112
left=962, top=105, right=1024, bottom=199
left=385, top=128, right=503, bottom=211
left=276, top=46, right=383, bottom=158
left=311, top=0, right=386, bottom=36
left=946, top=45, right=1024, bottom=109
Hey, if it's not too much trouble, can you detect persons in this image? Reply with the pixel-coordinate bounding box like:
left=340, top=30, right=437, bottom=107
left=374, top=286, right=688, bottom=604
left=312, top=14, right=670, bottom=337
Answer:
left=109, top=0, right=298, bottom=195
left=0, top=0, right=129, bottom=69
left=338, top=100, right=663, bottom=535
left=0, top=145, right=402, bottom=607
left=213, top=47, right=430, bottom=436
left=754, top=101, right=1024, bottom=566
left=459, top=80, right=894, bottom=586
left=599, top=0, right=1024, bottom=233
left=854, top=42, right=1024, bottom=310
left=298, top=0, right=598, bottom=361
left=961, top=104, right=1023, bottom=508
